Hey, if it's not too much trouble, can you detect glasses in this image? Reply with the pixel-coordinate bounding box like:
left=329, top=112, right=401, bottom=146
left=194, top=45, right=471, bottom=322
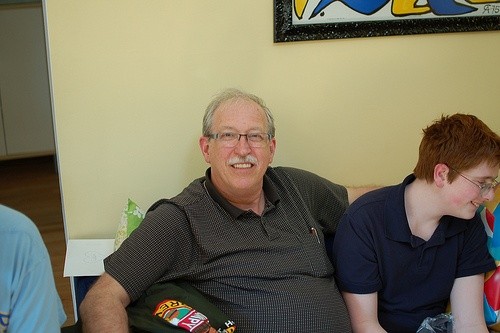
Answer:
left=433, top=162, right=500, bottom=197
left=204, top=131, right=271, bottom=148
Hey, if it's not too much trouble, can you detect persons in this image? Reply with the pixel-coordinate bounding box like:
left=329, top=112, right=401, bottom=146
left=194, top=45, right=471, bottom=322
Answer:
left=0, top=204, right=68, bottom=333
left=323, top=113, right=500, bottom=333
left=79, top=86, right=384, bottom=333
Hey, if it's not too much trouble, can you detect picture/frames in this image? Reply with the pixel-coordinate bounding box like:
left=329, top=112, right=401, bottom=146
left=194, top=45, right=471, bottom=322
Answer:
left=272, top=0, right=500, bottom=42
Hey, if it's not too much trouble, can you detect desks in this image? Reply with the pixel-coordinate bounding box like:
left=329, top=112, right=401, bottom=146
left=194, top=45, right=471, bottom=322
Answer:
left=64, top=239, right=115, bottom=322
